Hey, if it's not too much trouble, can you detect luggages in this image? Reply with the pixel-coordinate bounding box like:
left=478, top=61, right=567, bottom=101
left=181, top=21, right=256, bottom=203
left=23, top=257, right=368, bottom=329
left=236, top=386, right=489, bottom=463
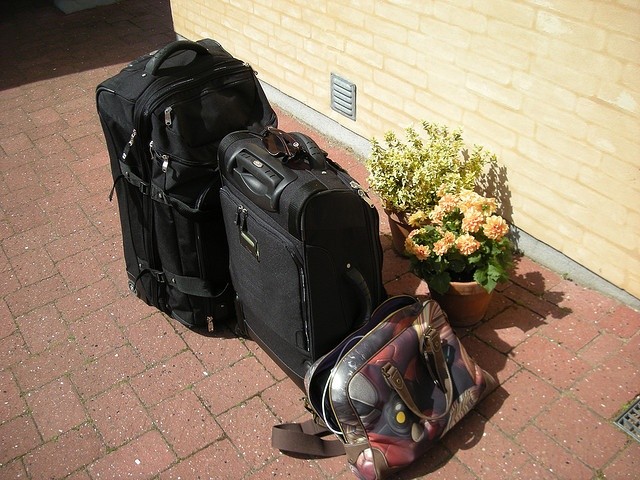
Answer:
left=92, top=39, right=278, bottom=332
left=216, top=124, right=381, bottom=413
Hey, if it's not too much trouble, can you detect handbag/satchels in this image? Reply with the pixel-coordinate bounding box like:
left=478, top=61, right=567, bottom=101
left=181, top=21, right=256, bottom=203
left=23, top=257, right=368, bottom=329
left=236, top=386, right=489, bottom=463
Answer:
left=272, top=292, right=500, bottom=480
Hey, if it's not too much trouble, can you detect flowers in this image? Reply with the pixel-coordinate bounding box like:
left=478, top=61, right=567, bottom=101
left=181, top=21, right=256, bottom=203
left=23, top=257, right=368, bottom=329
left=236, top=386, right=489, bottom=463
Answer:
left=369, top=118, right=505, bottom=221
left=405, top=189, right=510, bottom=291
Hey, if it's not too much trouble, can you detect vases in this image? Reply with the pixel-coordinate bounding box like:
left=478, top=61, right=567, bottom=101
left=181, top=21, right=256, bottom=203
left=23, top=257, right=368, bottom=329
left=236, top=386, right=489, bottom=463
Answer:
left=427, top=277, right=496, bottom=324
left=383, top=210, right=423, bottom=260
